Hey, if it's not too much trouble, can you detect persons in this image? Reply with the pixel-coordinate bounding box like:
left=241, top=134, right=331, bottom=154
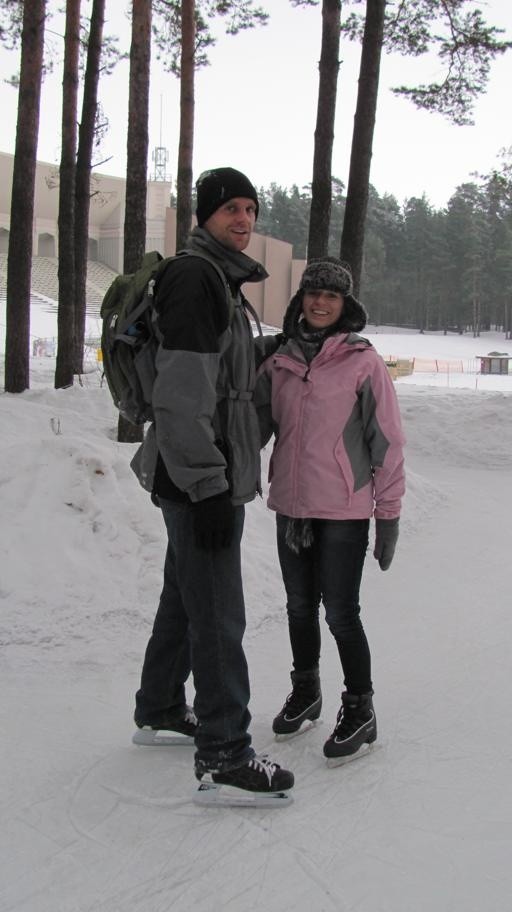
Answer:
left=128, top=166, right=297, bottom=795
left=248, top=259, right=406, bottom=762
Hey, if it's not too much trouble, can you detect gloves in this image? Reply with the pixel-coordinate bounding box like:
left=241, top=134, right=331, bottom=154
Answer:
left=372, top=516, right=401, bottom=571
left=199, top=495, right=239, bottom=543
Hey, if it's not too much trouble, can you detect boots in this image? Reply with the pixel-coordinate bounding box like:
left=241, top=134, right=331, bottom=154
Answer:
left=322, top=687, right=382, bottom=760
left=152, top=704, right=208, bottom=736
left=193, top=750, right=293, bottom=793
left=271, top=666, right=321, bottom=735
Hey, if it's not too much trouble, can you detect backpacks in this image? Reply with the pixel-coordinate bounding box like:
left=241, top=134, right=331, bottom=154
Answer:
left=98, top=240, right=170, bottom=443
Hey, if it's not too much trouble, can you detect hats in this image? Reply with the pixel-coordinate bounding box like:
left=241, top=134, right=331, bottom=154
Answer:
left=281, top=258, right=371, bottom=338
left=193, top=166, right=259, bottom=227
